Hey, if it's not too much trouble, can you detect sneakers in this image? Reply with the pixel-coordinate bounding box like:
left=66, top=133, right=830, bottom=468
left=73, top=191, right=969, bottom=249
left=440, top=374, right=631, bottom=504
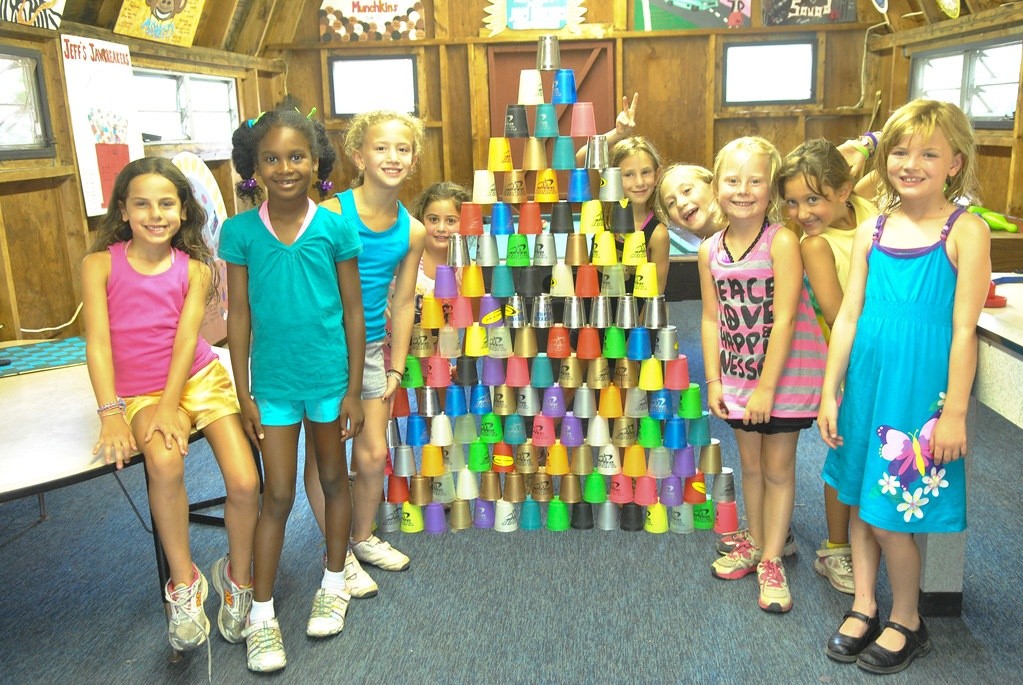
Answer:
left=307, top=579, right=351, bottom=637
left=716, top=527, right=797, bottom=556
left=814, top=539, right=855, bottom=594
left=350, top=534, right=410, bottom=571
left=325, top=546, right=378, bottom=598
left=756, top=557, right=793, bottom=612
left=165, top=562, right=211, bottom=682
left=240, top=612, right=286, bottom=672
left=212, top=556, right=254, bottom=644
left=710, top=534, right=761, bottom=579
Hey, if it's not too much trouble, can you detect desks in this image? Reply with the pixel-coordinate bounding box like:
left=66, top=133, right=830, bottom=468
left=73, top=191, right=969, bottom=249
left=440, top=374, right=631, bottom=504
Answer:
left=0, top=339, right=267, bottom=664
left=911, top=273, right=1023, bottom=618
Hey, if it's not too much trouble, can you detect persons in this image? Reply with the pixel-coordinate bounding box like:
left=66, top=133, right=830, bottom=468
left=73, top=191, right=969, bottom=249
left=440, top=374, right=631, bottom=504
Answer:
left=699, top=137, right=844, bottom=613
left=575, top=92, right=670, bottom=323
left=303, top=110, right=426, bottom=595
left=777, top=132, right=886, bottom=594
left=414, top=180, right=470, bottom=293
left=817, top=101, right=992, bottom=675
left=80, top=156, right=260, bottom=651
left=219, top=110, right=366, bottom=672
left=660, top=165, right=798, bottom=557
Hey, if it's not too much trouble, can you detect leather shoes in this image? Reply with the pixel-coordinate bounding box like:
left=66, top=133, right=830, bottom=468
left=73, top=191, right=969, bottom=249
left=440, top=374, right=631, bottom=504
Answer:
left=827, top=608, right=880, bottom=662
left=856, top=615, right=932, bottom=674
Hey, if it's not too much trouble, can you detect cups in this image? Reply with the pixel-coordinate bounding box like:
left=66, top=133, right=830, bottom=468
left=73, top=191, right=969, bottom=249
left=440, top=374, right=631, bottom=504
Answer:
left=371, top=34, right=738, bottom=535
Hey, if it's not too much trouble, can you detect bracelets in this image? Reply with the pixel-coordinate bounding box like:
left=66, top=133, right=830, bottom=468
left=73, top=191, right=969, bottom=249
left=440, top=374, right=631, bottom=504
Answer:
left=97, top=399, right=126, bottom=418
left=847, top=130, right=883, bottom=160
left=387, top=369, right=404, bottom=385
left=706, top=377, right=720, bottom=384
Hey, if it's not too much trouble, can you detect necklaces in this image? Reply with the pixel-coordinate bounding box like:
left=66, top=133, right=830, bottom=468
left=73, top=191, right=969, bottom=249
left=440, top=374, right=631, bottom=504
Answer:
left=722, top=219, right=766, bottom=263
